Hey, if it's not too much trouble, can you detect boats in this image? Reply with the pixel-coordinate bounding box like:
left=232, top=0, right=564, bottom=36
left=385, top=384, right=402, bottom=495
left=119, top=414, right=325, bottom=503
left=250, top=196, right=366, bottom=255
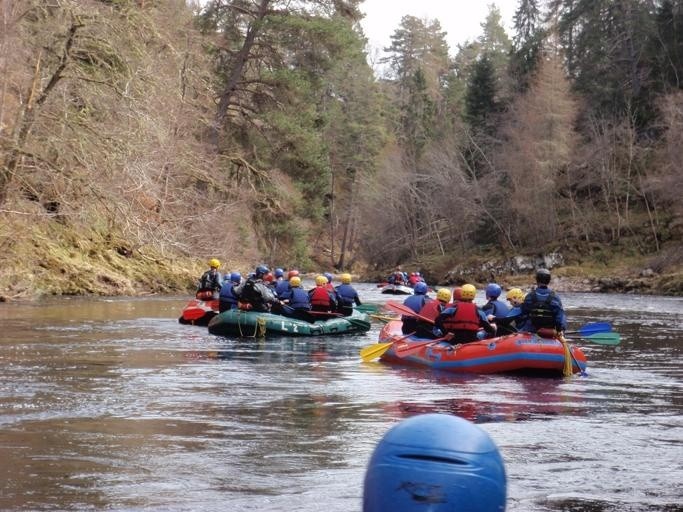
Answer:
left=208, top=310, right=370, bottom=339
left=380, top=284, right=438, bottom=297
left=379, top=317, right=589, bottom=378
left=179, top=298, right=254, bottom=324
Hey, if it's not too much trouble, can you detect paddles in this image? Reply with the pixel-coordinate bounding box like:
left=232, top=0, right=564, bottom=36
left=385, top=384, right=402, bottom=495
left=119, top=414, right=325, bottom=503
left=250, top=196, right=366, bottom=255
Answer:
left=564, top=322, right=612, bottom=336
left=394, top=337, right=449, bottom=358
left=385, top=300, right=435, bottom=323
left=339, top=316, right=370, bottom=332
left=565, top=333, right=621, bottom=345
left=343, top=304, right=379, bottom=315
left=285, top=304, right=316, bottom=324
left=360, top=331, right=416, bottom=362
left=569, top=350, right=588, bottom=377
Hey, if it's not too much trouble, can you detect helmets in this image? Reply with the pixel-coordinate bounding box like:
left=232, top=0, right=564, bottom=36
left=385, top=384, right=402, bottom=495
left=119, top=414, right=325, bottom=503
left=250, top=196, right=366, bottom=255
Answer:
left=507, top=287, right=524, bottom=305
left=315, top=270, right=353, bottom=285
left=289, top=267, right=303, bottom=287
left=224, top=265, right=285, bottom=283
left=485, top=283, right=501, bottom=299
left=534, top=268, right=551, bottom=286
left=436, top=287, right=451, bottom=303
left=390, top=264, right=420, bottom=277
left=208, top=257, right=219, bottom=269
left=359, top=411, right=508, bottom=511
left=415, top=280, right=427, bottom=294
left=452, top=284, right=476, bottom=300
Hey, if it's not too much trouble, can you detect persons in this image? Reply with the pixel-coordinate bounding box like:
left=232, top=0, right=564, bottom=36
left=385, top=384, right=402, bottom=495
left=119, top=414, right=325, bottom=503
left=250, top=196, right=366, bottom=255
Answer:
left=198, top=262, right=362, bottom=315
left=383, top=265, right=566, bottom=346
